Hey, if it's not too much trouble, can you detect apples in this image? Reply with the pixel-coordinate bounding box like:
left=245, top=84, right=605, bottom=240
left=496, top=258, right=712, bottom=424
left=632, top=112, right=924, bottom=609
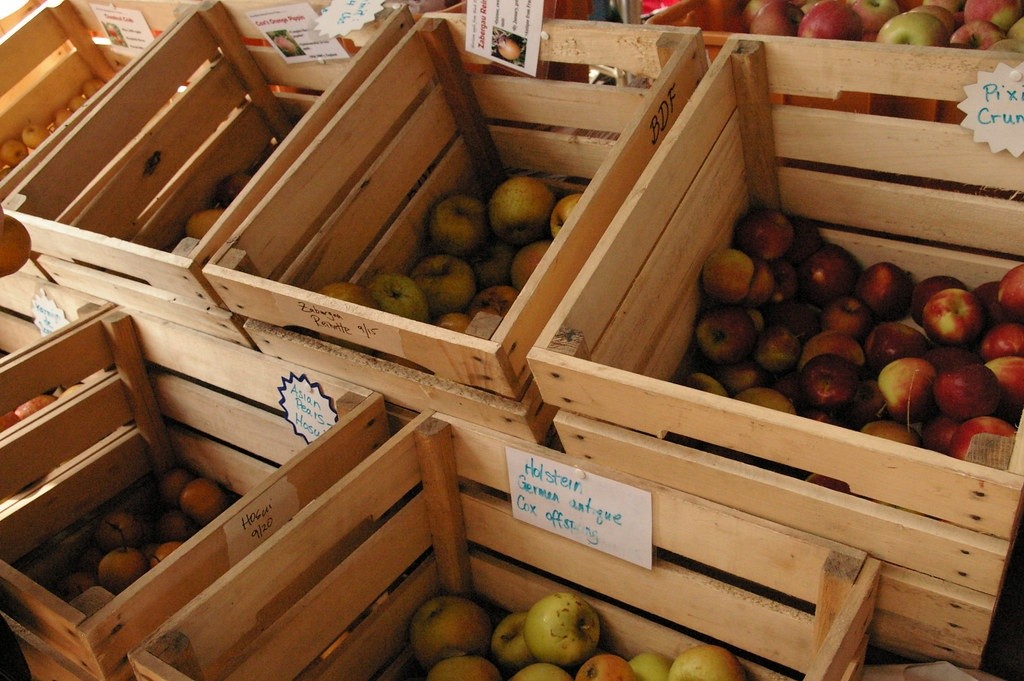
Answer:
left=408, top=590, right=749, bottom=681
left=311, top=175, right=586, bottom=375
left=497, top=37, right=521, bottom=60
left=0, top=382, right=83, bottom=434
left=734, top=0, right=1024, bottom=53
left=275, top=31, right=299, bottom=53
left=0, top=79, right=106, bottom=182
left=676, top=208, right=1024, bottom=492
left=182, top=174, right=253, bottom=239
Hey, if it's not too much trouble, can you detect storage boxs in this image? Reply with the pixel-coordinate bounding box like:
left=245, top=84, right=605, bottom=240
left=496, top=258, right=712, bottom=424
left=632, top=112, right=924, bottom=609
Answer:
left=0, top=0, right=1024, bottom=681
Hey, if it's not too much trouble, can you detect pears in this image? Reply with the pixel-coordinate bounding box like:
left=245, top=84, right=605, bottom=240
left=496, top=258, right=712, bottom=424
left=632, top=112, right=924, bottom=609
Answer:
left=54, top=467, right=225, bottom=603
